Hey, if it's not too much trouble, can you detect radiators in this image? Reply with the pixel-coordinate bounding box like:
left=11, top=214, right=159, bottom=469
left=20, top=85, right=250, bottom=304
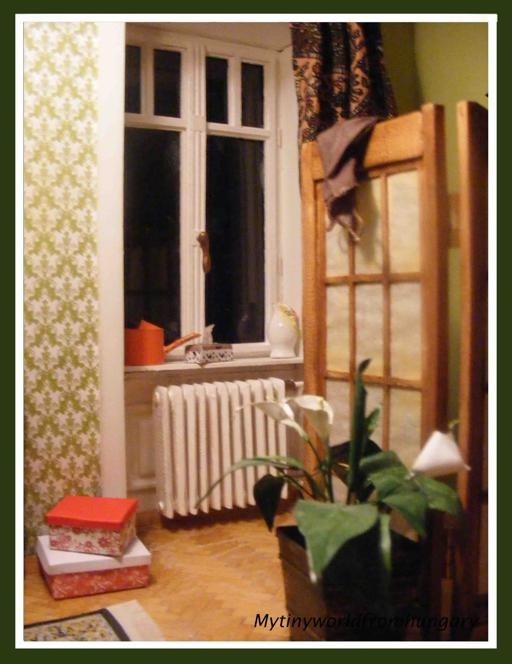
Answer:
left=150, top=376, right=290, bottom=520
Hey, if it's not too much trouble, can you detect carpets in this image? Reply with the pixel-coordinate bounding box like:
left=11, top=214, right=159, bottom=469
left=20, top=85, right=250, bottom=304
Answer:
left=25, top=599, right=167, bottom=641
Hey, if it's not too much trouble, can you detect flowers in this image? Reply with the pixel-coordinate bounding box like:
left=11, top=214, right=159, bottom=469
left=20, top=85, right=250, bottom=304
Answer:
left=194, top=358, right=470, bottom=588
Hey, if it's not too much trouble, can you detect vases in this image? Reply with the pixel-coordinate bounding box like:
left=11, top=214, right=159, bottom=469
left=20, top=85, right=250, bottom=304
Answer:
left=273, top=527, right=424, bottom=643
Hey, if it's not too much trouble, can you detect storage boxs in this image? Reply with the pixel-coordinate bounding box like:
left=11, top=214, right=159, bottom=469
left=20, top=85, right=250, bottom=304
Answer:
left=45, top=496, right=139, bottom=558
left=33, top=536, right=152, bottom=599
